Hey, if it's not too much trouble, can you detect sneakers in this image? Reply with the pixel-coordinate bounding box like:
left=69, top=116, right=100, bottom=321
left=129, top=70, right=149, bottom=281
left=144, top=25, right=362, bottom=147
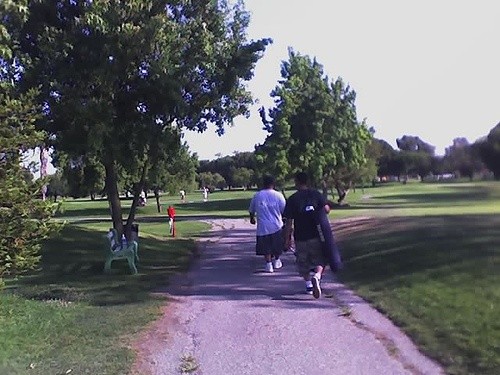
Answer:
left=306, top=284, right=314, bottom=294
left=265, top=262, right=273, bottom=272
left=273, top=259, right=283, bottom=268
left=312, top=275, right=321, bottom=298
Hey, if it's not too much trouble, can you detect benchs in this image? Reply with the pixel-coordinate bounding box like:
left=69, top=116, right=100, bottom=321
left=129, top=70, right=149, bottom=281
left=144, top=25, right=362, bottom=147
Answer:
left=102, top=228, right=140, bottom=275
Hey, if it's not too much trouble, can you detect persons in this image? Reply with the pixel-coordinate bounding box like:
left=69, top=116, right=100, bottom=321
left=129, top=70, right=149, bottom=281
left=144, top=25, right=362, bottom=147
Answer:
left=249, top=176, right=286, bottom=272
left=137, top=186, right=209, bottom=207
left=282, top=172, right=331, bottom=299
